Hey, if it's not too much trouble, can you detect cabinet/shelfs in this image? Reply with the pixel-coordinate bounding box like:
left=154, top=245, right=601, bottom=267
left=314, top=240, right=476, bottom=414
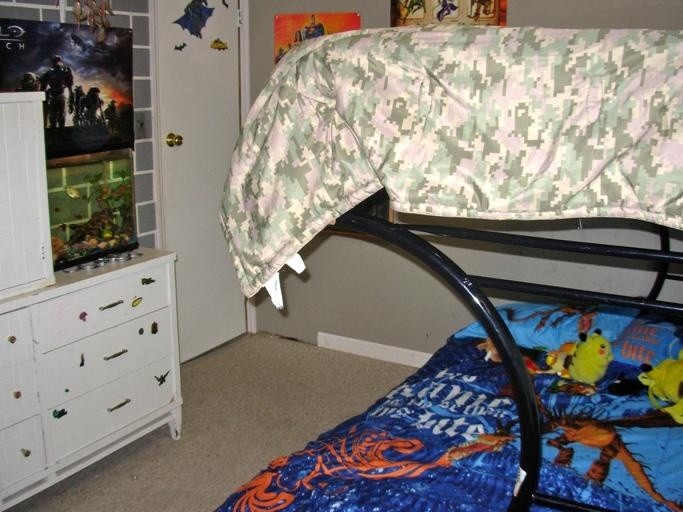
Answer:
left=0, top=89, right=55, bottom=303
left=0, top=249, right=185, bottom=511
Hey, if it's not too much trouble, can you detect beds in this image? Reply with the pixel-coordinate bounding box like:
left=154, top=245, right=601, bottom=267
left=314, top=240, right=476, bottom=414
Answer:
left=215, top=24, right=682, bottom=512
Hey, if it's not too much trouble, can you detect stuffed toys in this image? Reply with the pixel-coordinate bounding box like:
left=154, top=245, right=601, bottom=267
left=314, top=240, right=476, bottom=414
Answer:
left=566, top=327, right=611, bottom=386
left=474, top=334, right=501, bottom=364
left=638, top=356, right=682, bottom=404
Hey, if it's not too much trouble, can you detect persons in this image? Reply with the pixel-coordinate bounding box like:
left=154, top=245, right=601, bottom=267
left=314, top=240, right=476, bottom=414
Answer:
left=14, top=55, right=121, bottom=132
left=290, top=30, right=304, bottom=48
left=304, top=15, right=323, bottom=39
